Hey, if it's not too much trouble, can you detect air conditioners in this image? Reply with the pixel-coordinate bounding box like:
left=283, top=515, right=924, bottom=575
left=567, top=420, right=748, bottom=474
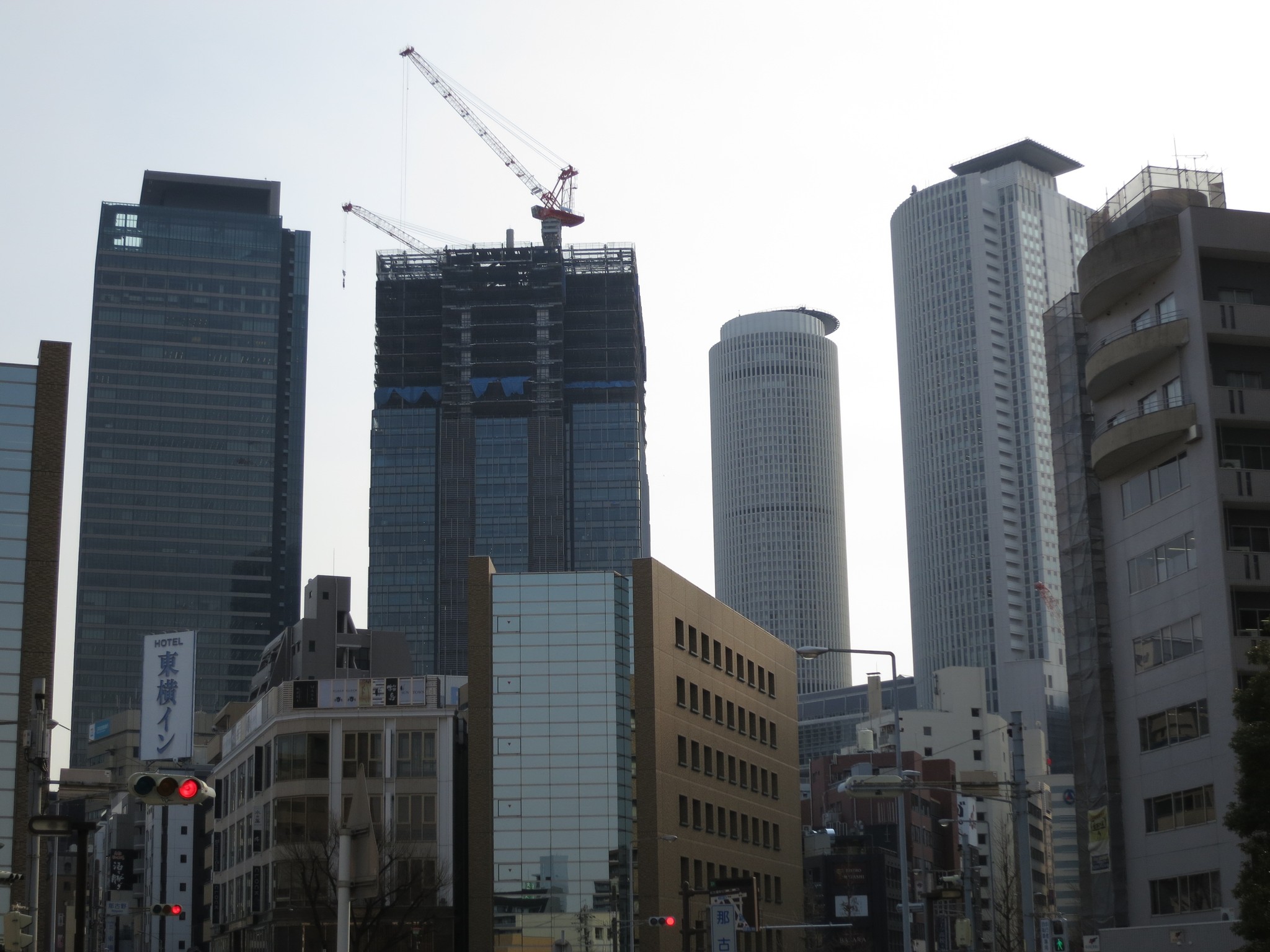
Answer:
left=803, top=825, right=812, bottom=831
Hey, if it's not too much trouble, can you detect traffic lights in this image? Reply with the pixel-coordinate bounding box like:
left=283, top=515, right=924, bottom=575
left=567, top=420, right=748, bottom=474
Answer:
left=1051, top=918, right=1070, bottom=952
left=127, top=772, right=204, bottom=802
left=0, top=869, right=24, bottom=887
left=150, top=904, right=183, bottom=916
left=942, top=875, right=960, bottom=887
left=648, top=916, right=676, bottom=927
left=4, top=911, right=33, bottom=952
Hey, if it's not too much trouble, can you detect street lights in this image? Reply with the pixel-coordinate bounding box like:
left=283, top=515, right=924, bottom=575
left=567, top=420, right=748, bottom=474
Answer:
left=937, top=818, right=998, bottom=952
left=902, top=769, right=931, bottom=952
left=795, top=645, right=914, bottom=952
left=629, top=835, right=679, bottom=952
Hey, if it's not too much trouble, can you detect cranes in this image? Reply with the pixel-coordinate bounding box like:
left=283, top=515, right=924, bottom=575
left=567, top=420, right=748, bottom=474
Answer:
left=398, top=44, right=585, bottom=247
left=341, top=200, right=444, bottom=263
